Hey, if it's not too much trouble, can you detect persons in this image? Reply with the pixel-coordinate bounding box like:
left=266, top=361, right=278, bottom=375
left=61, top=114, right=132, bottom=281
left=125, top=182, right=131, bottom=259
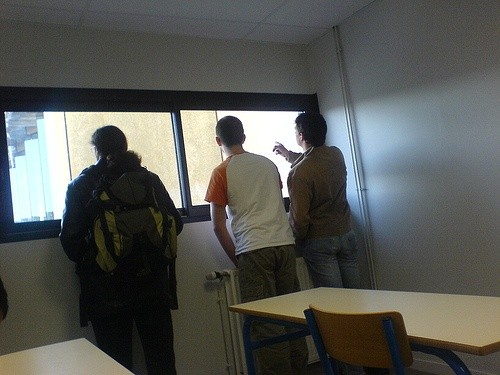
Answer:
left=204, top=116, right=309, bottom=375
left=60, top=125, right=184, bottom=375
left=273, top=110, right=367, bottom=375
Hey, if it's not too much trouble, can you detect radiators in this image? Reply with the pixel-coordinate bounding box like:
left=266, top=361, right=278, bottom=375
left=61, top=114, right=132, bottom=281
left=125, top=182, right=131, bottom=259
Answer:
left=206, top=255, right=330, bottom=375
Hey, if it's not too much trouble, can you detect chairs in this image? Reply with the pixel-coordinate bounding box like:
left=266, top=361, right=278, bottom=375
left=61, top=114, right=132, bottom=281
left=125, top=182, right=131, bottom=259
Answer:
left=302, top=299, right=417, bottom=375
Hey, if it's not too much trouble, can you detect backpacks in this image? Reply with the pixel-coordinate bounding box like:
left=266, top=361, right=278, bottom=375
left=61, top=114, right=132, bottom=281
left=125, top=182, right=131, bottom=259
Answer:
left=80, top=168, right=177, bottom=277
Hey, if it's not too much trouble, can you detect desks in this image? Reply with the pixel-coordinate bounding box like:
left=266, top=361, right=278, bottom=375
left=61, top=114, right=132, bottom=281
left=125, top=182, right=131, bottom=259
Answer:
left=229, top=284, right=500, bottom=375
left=1, top=338, right=135, bottom=375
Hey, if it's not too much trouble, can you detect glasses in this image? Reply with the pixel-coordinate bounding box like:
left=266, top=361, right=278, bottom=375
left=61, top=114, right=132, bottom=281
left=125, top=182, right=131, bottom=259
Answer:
left=90, top=145, right=96, bottom=153
